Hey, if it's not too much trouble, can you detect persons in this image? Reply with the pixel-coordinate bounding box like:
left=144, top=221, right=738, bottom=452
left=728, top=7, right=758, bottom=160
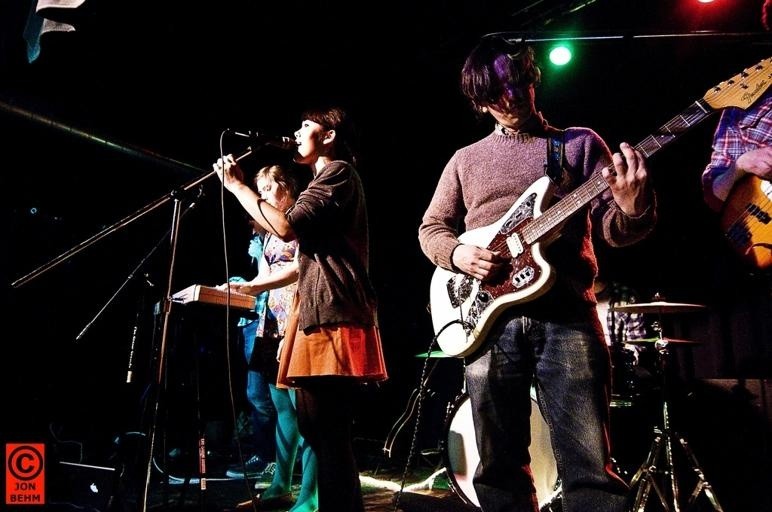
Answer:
left=701, top=0, right=772, bottom=379
left=225, top=226, right=280, bottom=492
left=418, top=35, right=658, bottom=512
left=253, top=164, right=321, bottom=512
left=213, top=104, right=390, bottom=512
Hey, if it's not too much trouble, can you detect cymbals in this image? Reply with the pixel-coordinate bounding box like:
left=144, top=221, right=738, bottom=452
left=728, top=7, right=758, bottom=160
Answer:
left=609, top=300, right=705, bottom=313
left=416, top=350, right=443, bottom=358
left=620, top=336, right=697, bottom=346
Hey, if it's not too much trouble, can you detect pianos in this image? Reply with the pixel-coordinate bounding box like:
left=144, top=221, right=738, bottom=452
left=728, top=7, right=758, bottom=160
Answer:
left=153, top=284, right=256, bottom=317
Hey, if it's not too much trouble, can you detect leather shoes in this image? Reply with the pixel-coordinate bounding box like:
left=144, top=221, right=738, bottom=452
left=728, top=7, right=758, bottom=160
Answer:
left=235, top=493, right=295, bottom=512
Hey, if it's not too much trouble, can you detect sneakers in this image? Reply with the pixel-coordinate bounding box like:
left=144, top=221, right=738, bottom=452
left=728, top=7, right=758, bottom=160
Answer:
left=226, top=454, right=267, bottom=478
left=254, top=462, right=276, bottom=489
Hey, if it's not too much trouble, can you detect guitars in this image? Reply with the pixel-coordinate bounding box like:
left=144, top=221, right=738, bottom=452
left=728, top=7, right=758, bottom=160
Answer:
left=429, top=54, right=771, bottom=358
left=722, top=174, right=771, bottom=271
left=382, top=358, right=441, bottom=466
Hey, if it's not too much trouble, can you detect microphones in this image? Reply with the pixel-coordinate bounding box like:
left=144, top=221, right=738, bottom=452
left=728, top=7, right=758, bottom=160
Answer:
left=247, top=129, right=294, bottom=150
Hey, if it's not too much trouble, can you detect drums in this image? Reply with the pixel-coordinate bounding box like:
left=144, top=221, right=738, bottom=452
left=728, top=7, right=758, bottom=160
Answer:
left=440, top=378, right=562, bottom=512
left=606, top=393, right=643, bottom=475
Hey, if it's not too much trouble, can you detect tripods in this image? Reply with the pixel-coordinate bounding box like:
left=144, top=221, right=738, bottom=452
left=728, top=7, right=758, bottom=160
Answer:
left=632, top=315, right=724, bottom=512
